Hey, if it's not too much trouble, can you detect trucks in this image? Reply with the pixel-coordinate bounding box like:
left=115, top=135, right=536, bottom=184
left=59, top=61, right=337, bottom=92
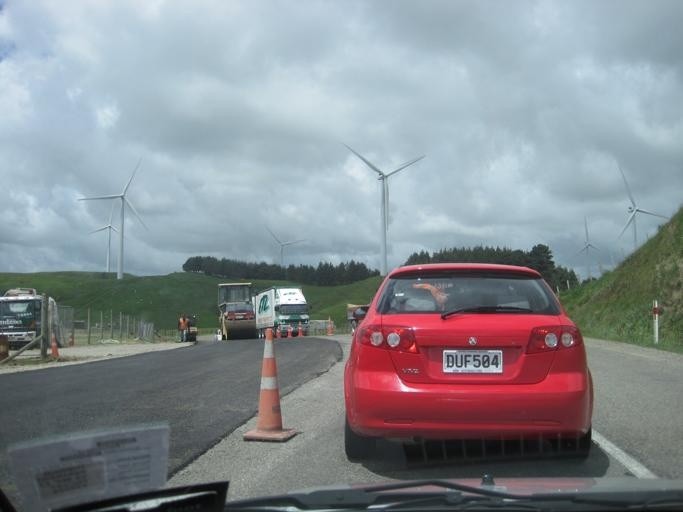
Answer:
left=0, top=287, right=60, bottom=350
left=251, top=285, right=311, bottom=339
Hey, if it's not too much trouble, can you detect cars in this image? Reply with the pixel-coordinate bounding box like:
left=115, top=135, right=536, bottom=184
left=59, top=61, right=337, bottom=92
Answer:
left=341, top=261, right=596, bottom=463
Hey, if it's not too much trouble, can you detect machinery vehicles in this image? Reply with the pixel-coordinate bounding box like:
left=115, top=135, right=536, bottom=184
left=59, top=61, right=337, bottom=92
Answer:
left=216, top=282, right=257, bottom=340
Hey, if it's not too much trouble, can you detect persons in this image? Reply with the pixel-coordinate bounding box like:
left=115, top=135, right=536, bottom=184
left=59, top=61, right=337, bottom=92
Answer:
left=177, top=312, right=189, bottom=343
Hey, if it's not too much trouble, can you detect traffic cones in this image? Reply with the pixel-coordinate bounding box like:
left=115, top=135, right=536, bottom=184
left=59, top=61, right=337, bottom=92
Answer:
left=326, top=315, right=334, bottom=336
left=296, top=320, right=304, bottom=337
left=275, top=324, right=282, bottom=339
left=285, top=323, right=293, bottom=338
left=241, top=326, right=299, bottom=445
left=48, top=332, right=60, bottom=357
